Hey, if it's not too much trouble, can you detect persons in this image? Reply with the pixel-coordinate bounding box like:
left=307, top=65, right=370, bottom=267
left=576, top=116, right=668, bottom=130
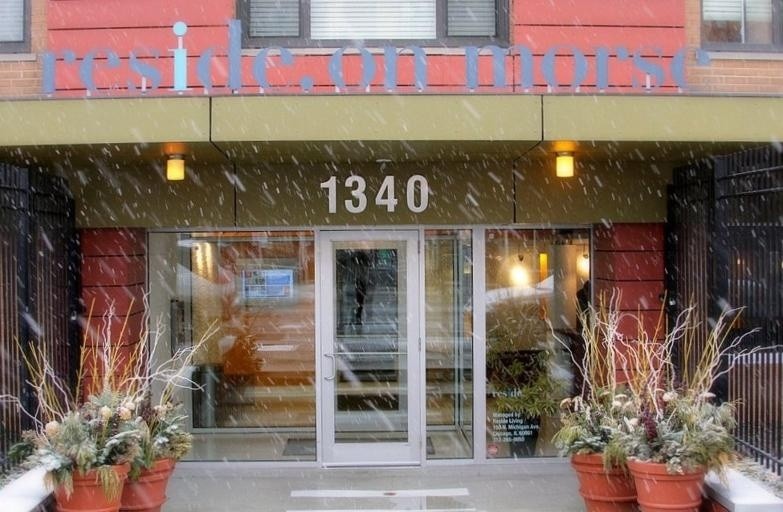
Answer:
left=348, top=276, right=366, bottom=326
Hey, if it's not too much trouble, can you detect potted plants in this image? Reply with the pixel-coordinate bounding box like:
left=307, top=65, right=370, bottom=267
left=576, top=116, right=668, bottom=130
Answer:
left=486, top=291, right=559, bottom=456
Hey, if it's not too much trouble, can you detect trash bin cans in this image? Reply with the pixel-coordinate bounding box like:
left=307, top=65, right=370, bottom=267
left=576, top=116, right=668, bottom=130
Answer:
left=190, top=363, right=222, bottom=429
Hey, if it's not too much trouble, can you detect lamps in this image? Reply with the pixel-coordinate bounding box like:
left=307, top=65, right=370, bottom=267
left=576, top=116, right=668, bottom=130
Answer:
left=164, top=155, right=186, bottom=181
left=554, top=151, right=574, bottom=178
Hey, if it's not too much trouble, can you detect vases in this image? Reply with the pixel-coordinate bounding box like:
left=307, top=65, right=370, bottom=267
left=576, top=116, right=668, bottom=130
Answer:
left=571, top=451, right=705, bottom=512
left=50, top=458, right=174, bottom=512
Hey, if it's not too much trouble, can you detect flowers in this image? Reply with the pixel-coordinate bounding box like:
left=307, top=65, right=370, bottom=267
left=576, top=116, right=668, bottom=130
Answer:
left=34, top=391, right=194, bottom=500
left=550, top=387, right=737, bottom=485
